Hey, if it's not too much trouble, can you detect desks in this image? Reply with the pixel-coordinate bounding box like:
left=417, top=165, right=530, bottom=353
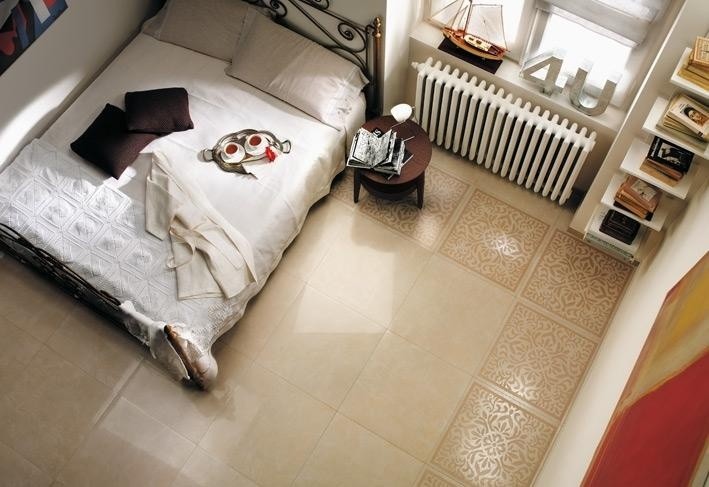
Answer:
left=353, top=115, right=432, bottom=209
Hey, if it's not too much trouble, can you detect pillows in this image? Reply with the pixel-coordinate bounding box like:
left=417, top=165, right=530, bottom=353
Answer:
left=70, top=103, right=171, bottom=180
left=224, top=6, right=370, bottom=132
left=124, top=88, right=193, bottom=134
left=141, top=0, right=268, bottom=63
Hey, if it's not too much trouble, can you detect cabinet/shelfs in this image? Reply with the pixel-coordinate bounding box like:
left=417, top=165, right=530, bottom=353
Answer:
left=585, top=48, right=709, bottom=271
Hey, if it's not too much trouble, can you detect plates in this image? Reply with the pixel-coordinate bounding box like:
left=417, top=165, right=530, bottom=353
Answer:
left=221, top=145, right=245, bottom=164
left=243, top=139, right=269, bottom=156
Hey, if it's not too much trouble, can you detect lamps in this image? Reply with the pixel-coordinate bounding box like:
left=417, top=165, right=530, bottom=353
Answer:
left=391, top=103, right=415, bottom=125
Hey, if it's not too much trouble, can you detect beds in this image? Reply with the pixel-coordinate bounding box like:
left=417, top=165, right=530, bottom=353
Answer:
left=0, top=0, right=381, bottom=391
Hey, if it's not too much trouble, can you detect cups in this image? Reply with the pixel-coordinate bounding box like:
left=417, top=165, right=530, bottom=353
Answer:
left=247, top=133, right=263, bottom=151
left=223, top=142, right=240, bottom=159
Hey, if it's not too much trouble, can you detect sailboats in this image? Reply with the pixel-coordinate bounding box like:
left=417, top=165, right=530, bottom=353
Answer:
left=442, top=0, right=512, bottom=66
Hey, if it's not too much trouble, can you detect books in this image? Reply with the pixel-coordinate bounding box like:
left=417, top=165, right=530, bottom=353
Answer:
left=346, top=127, right=414, bottom=180
left=599, top=36, right=709, bottom=245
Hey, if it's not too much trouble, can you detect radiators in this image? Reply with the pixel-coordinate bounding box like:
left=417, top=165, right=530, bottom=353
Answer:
left=410, top=56, right=596, bottom=206
